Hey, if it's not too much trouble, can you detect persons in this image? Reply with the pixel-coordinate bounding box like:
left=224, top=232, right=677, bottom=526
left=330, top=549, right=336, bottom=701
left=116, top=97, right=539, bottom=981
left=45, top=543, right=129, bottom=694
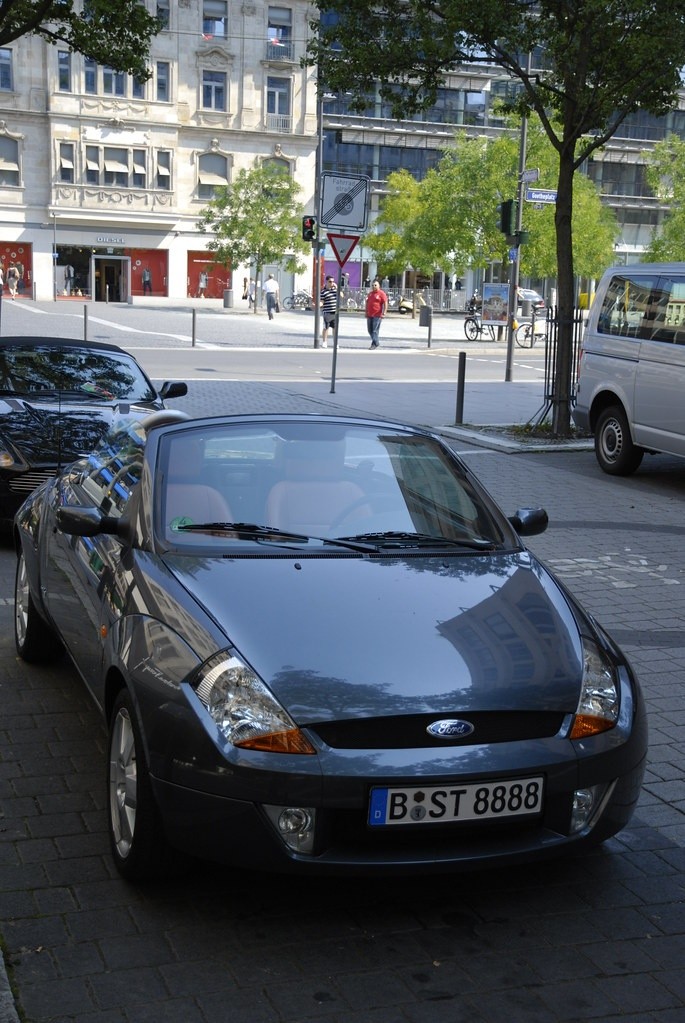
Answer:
left=65, top=264, right=74, bottom=291
left=320, top=276, right=339, bottom=349
left=0, top=262, right=20, bottom=300
left=15, top=262, right=24, bottom=294
left=244, top=277, right=255, bottom=308
left=198, top=272, right=208, bottom=297
left=142, top=268, right=152, bottom=296
left=448, top=278, right=463, bottom=290
left=366, top=280, right=388, bottom=350
left=263, top=274, right=279, bottom=320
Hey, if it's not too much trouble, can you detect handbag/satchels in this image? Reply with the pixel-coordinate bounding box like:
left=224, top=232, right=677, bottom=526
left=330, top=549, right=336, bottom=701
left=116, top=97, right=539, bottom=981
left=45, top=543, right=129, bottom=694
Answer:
left=275, top=302, right=282, bottom=313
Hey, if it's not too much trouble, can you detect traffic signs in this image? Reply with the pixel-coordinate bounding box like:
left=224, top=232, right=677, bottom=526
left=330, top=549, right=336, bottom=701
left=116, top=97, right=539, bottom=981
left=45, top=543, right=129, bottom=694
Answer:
left=525, top=188, right=557, bottom=205
left=523, top=168, right=538, bottom=183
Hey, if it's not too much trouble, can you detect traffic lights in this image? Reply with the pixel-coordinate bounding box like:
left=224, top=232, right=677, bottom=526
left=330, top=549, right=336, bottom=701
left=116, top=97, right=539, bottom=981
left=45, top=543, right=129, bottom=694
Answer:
left=302, top=215, right=315, bottom=241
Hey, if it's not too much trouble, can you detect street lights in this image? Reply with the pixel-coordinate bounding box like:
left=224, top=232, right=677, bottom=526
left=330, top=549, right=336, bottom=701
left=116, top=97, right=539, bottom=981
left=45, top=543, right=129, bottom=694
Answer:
left=314, top=93, right=336, bottom=348
left=52, top=212, right=60, bottom=301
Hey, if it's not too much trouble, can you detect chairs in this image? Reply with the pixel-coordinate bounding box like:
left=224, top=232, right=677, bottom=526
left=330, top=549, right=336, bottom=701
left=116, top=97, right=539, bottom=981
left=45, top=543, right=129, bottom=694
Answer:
left=263, top=427, right=373, bottom=535
left=166, top=432, right=240, bottom=542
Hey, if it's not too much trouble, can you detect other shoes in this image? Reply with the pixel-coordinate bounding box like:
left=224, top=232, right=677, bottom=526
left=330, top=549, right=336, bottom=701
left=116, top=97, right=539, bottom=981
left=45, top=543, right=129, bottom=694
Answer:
left=369, top=345, right=377, bottom=350
left=321, top=341, right=327, bottom=349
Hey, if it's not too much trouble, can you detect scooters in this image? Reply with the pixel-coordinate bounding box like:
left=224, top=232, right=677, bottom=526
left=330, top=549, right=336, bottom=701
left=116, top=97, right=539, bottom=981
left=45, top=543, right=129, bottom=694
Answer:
left=398, top=288, right=426, bottom=314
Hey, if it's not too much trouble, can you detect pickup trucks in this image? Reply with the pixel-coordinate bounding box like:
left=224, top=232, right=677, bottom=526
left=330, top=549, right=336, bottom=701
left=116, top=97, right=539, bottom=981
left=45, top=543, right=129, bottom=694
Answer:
left=609, top=301, right=645, bottom=328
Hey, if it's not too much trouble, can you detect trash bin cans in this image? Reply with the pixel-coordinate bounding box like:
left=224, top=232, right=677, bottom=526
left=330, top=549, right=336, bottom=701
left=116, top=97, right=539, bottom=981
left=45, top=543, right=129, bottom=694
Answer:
left=580, top=291, right=594, bottom=310
left=522, top=299, right=531, bottom=316
left=419, top=305, right=433, bottom=327
left=223, top=289, right=233, bottom=308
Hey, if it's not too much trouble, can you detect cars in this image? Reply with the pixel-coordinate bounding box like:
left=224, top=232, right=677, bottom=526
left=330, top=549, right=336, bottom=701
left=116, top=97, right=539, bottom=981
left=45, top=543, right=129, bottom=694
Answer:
left=14, top=410, right=647, bottom=889
left=0, top=337, right=188, bottom=544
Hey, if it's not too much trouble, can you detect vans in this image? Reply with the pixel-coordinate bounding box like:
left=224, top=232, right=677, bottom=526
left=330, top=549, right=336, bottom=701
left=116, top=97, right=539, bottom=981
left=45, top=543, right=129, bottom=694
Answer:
left=572, top=262, right=685, bottom=474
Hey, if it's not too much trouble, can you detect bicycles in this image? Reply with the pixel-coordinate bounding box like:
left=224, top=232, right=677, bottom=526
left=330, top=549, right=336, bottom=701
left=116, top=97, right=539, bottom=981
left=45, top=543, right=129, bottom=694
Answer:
left=282, top=292, right=311, bottom=310
left=464, top=304, right=496, bottom=342
left=515, top=302, right=557, bottom=349
